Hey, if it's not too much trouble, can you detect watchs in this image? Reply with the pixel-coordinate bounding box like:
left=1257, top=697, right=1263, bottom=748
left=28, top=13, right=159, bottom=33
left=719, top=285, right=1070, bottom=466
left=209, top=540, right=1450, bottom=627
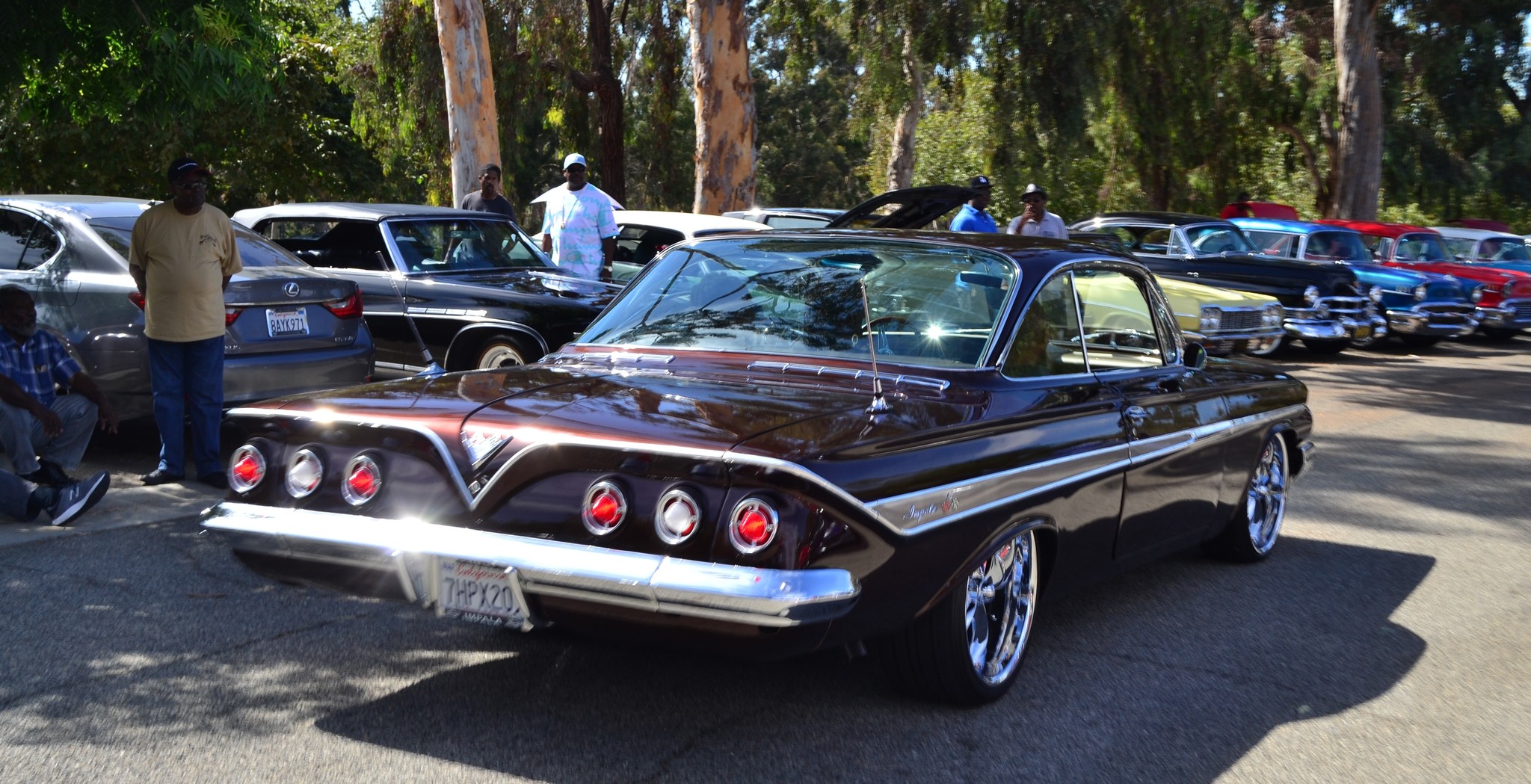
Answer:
left=603, top=265, right=613, bottom=272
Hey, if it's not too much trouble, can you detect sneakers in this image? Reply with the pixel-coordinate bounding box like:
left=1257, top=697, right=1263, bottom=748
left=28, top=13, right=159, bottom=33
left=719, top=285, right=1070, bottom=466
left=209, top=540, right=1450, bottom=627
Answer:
left=47, top=469, right=111, bottom=526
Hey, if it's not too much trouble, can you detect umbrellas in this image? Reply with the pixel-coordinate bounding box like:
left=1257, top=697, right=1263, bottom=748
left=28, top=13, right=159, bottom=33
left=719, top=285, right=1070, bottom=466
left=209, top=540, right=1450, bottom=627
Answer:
left=531, top=180, right=626, bottom=211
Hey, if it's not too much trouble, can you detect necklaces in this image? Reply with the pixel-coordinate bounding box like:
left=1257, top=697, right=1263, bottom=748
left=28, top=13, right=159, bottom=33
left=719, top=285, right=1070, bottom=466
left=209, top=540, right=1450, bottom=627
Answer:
left=561, top=187, right=582, bottom=229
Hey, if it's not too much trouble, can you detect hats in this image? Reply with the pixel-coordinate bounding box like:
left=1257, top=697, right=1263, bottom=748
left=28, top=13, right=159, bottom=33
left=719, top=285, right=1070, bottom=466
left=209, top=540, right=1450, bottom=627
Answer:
left=563, top=153, right=586, bottom=169
left=970, top=175, right=994, bottom=192
left=167, top=159, right=210, bottom=184
left=1020, top=183, right=1047, bottom=202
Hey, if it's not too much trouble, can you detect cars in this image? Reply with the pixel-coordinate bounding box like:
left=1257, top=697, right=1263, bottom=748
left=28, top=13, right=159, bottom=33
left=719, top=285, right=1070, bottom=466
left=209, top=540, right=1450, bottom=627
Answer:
left=1067, top=212, right=1390, bottom=359
left=720, top=184, right=1531, bottom=356
left=506, top=210, right=822, bottom=315
left=0, top=193, right=375, bottom=433
left=230, top=200, right=691, bottom=380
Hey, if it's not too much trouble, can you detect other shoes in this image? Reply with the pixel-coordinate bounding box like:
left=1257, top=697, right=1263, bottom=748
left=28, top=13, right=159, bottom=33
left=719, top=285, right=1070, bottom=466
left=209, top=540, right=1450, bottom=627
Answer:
left=139, top=469, right=186, bottom=484
left=201, top=471, right=228, bottom=488
left=15, top=459, right=83, bottom=486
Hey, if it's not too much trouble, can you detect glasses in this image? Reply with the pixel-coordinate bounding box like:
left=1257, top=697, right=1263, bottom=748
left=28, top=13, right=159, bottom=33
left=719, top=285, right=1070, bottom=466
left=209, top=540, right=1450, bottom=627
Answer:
left=1023, top=199, right=1040, bottom=204
left=175, top=181, right=207, bottom=190
left=566, top=167, right=585, bottom=173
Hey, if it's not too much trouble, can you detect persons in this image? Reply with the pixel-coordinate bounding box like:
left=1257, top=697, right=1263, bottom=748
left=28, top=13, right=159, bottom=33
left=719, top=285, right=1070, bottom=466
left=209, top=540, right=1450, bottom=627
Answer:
left=1006, top=183, right=1070, bottom=328
left=0, top=284, right=119, bottom=486
left=1221, top=192, right=1250, bottom=218
left=443, top=163, right=520, bottom=271
left=950, top=175, right=1006, bottom=323
left=129, top=158, right=242, bottom=486
left=0, top=470, right=110, bottom=526
left=539, top=152, right=620, bottom=284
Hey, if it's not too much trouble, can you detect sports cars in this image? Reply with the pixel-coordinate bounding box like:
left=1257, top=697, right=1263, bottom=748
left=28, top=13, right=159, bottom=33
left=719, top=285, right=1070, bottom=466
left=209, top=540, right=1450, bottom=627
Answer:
left=193, top=228, right=1313, bottom=711
